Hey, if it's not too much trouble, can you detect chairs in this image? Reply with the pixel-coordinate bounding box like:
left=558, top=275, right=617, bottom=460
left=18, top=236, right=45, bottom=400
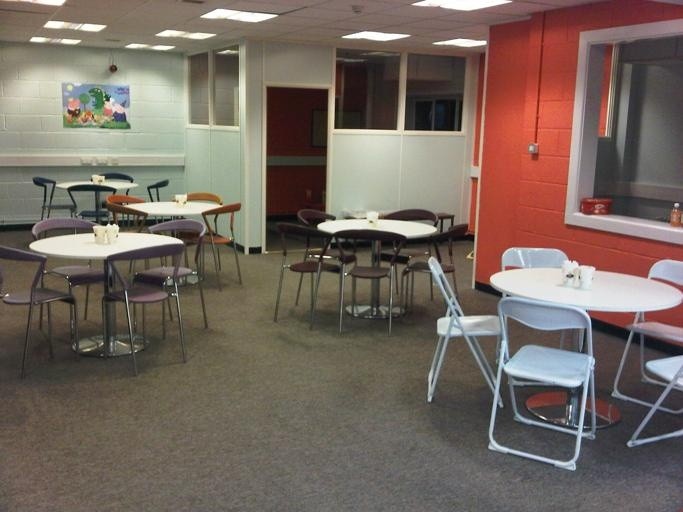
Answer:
left=427, top=257, right=507, bottom=409
left=497, top=247, right=575, bottom=363
left=610, top=259, right=683, bottom=414
left=272, top=209, right=468, bottom=335
left=625, top=355, right=683, bottom=449
left=489, top=298, right=597, bottom=471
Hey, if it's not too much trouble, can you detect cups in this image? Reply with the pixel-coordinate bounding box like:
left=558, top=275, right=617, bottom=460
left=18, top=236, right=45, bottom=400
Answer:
left=93, top=224, right=120, bottom=243
left=367, top=212, right=378, bottom=226
left=175, top=194, right=187, bottom=204
left=91, top=174, right=105, bottom=186
left=581, top=266, right=594, bottom=289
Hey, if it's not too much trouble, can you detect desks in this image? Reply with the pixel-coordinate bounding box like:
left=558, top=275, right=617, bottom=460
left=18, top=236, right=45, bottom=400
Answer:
left=489, top=266, right=683, bottom=430
